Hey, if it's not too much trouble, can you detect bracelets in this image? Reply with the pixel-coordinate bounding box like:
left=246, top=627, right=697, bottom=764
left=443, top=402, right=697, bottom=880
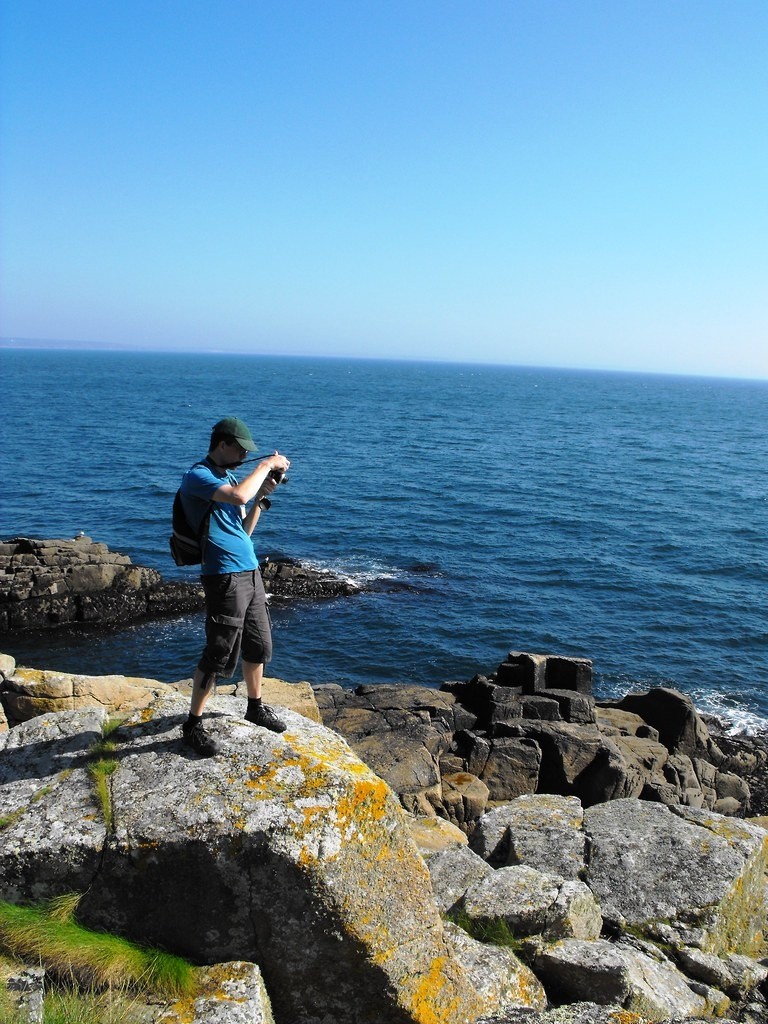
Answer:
left=254, top=496, right=272, bottom=511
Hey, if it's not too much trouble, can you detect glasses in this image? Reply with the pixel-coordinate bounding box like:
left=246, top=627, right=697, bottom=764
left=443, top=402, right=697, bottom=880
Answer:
left=232, top=439, right=249, bottom=456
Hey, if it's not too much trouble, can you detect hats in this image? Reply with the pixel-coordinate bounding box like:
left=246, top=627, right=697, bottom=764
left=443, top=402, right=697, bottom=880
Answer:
left=212, top=416, right=259, bottom=452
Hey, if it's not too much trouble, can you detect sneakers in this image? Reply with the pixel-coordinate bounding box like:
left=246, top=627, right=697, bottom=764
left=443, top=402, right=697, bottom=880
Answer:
left=183, top=721, right=220, bottom=757
left=244, top=705, right=287, bottom=733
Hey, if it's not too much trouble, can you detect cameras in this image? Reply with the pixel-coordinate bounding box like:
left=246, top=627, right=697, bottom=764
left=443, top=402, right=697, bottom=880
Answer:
left=270, top=469, right=289, bottom=485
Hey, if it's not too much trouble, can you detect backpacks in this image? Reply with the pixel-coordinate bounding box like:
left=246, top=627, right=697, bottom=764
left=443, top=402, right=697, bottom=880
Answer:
left=170, top=462, right=236, bottom=567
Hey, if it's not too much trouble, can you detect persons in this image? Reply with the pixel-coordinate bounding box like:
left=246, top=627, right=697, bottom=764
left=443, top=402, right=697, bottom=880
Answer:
left=180, top=418, right=290, bottom=757
